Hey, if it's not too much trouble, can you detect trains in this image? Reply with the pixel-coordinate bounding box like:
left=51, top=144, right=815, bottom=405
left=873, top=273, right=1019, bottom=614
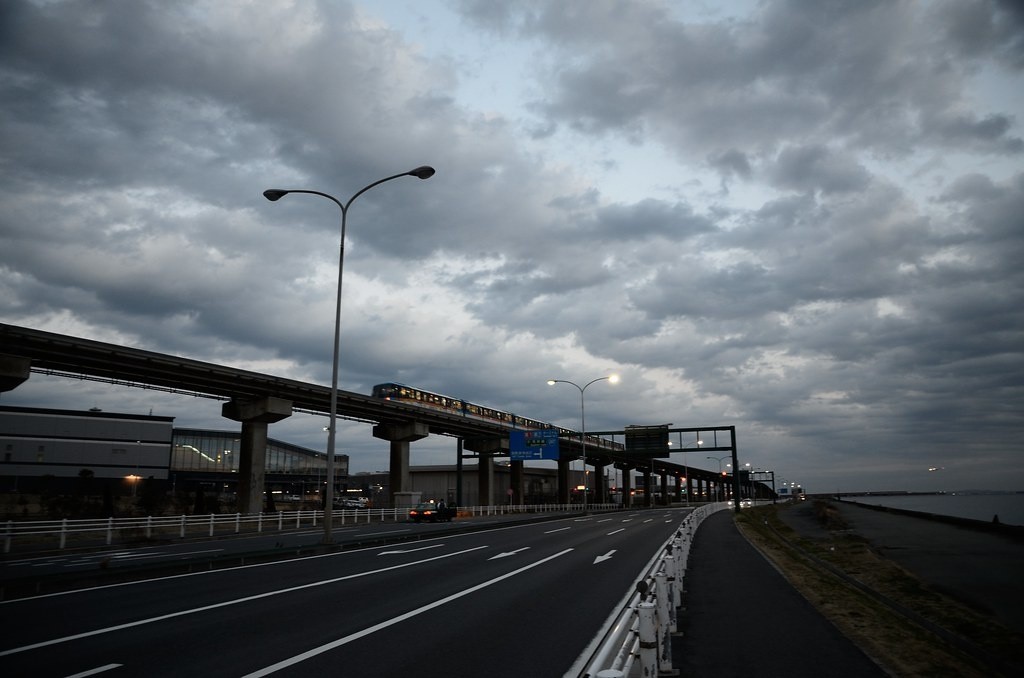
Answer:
left=370, top=381, right=626, bottom=456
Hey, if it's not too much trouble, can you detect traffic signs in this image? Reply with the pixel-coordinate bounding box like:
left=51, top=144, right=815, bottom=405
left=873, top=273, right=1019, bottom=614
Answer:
left=509, top=428, right=560, bottom=459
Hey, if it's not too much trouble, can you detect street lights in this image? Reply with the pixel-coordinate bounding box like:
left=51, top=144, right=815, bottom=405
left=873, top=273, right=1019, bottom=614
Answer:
left=668, top=441, right=703, bottom=507
left=547, top=374, right=619, bottom=512
left=134, top=441, right=140, bottom=496
left=263, top=164, right=436, bottom=531
left=315, top=454, right=321, bottom=505
left=706, top=456, right=732, bottom=501
left=751, top=467, right=769, bottom=501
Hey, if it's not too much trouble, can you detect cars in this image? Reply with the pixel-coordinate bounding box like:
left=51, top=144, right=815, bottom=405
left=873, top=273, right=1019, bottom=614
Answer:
left=289, top=495, right=300, bottom=501
left=635, top=490, right=644, bottom=498
left=409, top=501, right=458, bottom=523
left=333, top=496, right=368, bottom=509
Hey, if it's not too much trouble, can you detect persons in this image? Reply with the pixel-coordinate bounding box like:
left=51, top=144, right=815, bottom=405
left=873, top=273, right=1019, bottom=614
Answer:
left=438, top=499, right=447, bottom=522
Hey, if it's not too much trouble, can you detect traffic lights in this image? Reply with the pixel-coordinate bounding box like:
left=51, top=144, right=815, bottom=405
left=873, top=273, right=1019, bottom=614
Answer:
left=586, top=487, right=590, bottom=492
left=573, top=486, right=577, bottom=492
